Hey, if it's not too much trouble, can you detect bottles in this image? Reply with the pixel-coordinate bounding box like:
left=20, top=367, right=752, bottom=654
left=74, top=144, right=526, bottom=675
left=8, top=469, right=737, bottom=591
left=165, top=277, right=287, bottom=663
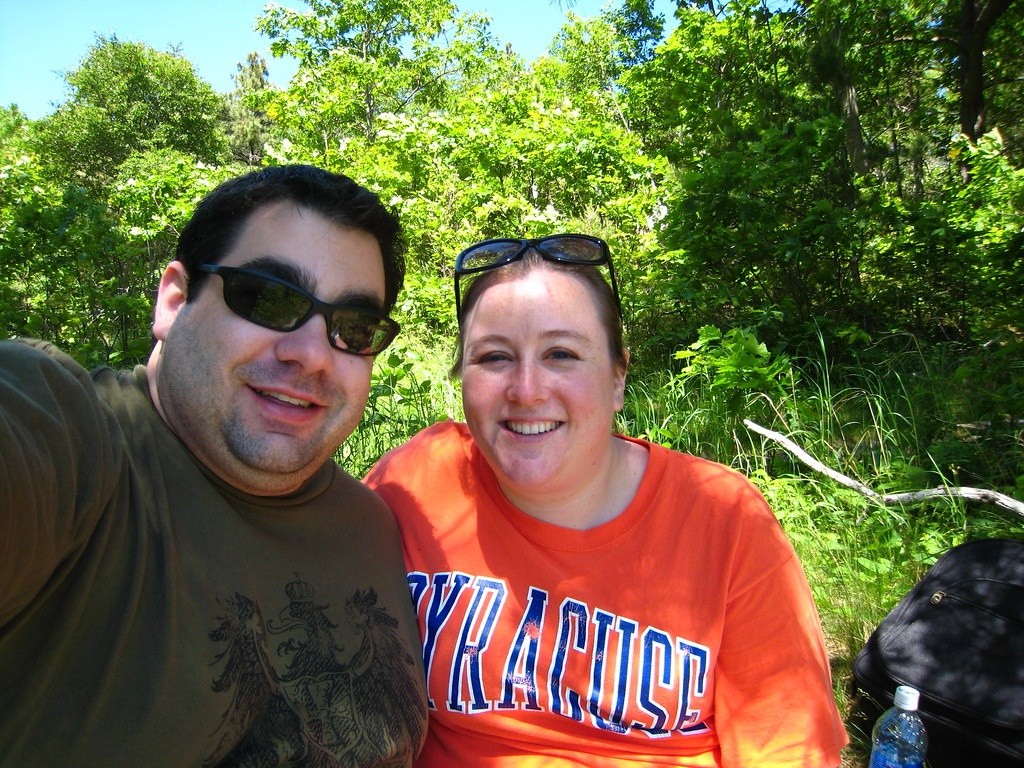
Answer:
left=867, top=685, right=928, bottom=768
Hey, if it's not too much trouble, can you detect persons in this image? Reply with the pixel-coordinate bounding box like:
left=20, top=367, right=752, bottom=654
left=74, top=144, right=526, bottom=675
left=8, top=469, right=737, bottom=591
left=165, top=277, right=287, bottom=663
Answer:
left=0, top=165, right=429, bottom=768
left=361, top=235, right=851, bottom=768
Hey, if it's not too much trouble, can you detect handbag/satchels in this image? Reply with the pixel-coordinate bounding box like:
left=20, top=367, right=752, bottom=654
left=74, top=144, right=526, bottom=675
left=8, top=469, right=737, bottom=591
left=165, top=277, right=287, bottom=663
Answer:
left=853, top=538, right=1024, bottom=768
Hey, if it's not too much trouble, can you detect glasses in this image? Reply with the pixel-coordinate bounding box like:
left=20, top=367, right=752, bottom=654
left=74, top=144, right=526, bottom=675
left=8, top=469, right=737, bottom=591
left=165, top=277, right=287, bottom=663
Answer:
left=199, top=263, right=401, bottom=356
left=454, top=233, right=622, bottom=327
